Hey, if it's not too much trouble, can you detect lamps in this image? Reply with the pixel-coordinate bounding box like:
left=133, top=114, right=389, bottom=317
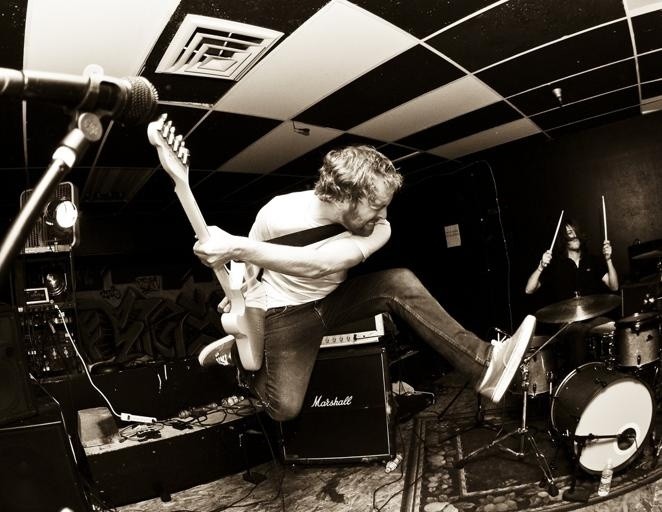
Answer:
left=44, top=196, right=79, bottom=233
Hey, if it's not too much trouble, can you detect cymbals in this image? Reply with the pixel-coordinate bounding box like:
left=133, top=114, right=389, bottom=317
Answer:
left=536, top=292, right=622, bottom=325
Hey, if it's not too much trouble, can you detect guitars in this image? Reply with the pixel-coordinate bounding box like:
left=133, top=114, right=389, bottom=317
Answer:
left=148, top=113, right=264, bottom=372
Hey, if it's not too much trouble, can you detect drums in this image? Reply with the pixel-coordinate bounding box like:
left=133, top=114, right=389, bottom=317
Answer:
left=549, top=359, right=656, bottom=476
left=615, top=312, right=662, bottom=367
left=507, top=335, right=556, bottom=395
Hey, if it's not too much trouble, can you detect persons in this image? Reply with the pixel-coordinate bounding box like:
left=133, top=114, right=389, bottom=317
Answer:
left=525, top=217, right=619, bottom=307
left=192, top=145, right=537, bottom=422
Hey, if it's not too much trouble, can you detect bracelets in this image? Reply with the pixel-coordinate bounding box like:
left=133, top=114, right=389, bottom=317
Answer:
left=606, top=258, right=611, bottom=262
left=537, top=266, right=544, bottom=272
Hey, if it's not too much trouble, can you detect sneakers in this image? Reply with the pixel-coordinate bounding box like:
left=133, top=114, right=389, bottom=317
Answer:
left=478, top=314, right=536, bottom=403
left=198, top=334, right=236, bottom=366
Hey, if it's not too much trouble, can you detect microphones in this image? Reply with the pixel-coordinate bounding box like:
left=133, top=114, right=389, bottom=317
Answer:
left=1, top=68, right=159, bottom=123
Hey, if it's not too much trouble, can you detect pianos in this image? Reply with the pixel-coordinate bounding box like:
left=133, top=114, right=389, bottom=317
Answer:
left=621, top=240, right=661, bottom=296
